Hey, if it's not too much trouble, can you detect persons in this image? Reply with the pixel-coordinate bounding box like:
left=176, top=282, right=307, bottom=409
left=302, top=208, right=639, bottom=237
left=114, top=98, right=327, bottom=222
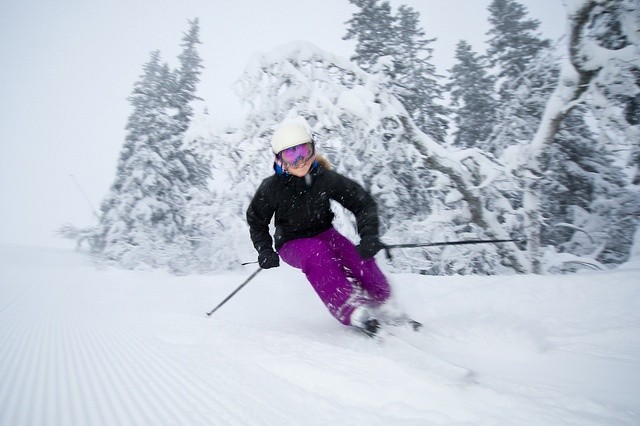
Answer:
left=244, top=113, right=406, bottom=334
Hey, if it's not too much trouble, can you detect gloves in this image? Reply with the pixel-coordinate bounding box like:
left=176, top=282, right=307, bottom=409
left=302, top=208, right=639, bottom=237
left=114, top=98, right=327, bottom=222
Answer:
left=360, top=238, right=382, bottom=259
left=259, top=247, right=279, bottom=269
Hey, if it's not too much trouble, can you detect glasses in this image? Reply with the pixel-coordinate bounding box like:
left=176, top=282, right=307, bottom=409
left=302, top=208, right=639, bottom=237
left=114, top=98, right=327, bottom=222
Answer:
left=276, top=143, right=315, bottom=165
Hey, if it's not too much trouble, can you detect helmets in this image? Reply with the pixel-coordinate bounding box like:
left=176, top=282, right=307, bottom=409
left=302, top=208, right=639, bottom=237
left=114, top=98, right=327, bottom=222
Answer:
left=270, top=115, right=314, bottom=154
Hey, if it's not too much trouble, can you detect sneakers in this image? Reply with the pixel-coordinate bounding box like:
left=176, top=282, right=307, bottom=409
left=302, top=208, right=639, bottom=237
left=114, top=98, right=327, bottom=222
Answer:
left=350, top=307, right=368, bottom=329
